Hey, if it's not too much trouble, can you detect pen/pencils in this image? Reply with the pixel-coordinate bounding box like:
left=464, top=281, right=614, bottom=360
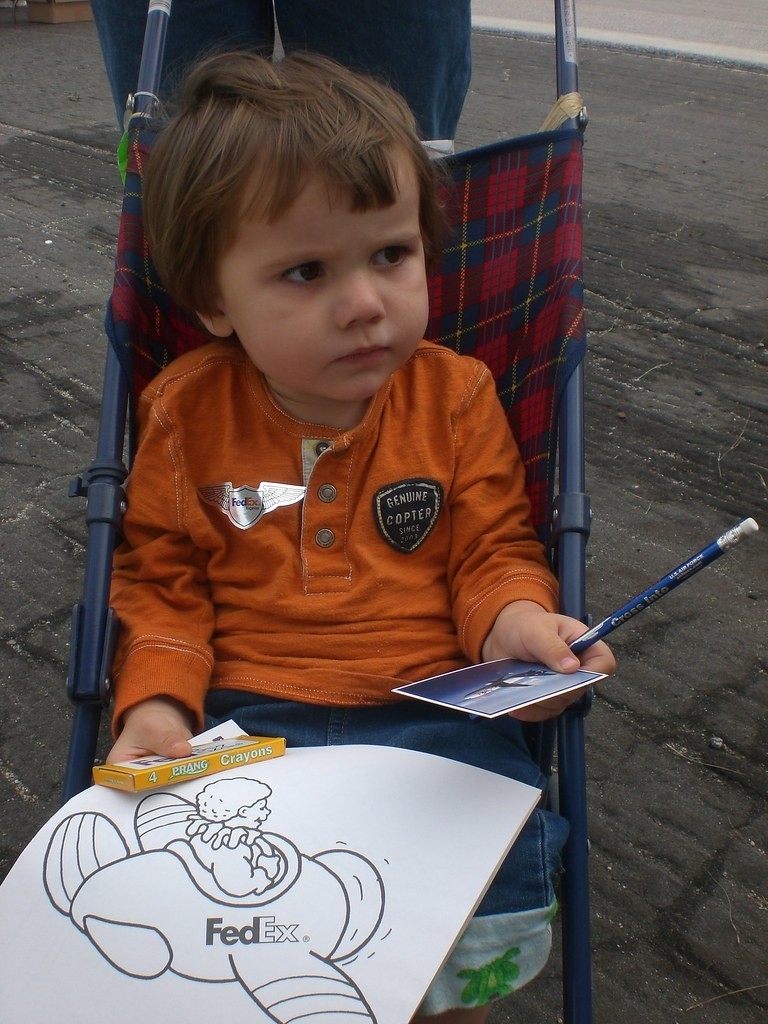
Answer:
left=564, top=517, right=760, bottom=656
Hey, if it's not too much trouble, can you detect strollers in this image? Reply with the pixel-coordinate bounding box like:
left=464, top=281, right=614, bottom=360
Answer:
left=63, top=0, right=596, bottom=1024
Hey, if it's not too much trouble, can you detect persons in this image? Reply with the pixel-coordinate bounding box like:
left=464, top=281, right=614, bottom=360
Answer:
left=105, top=55, right=617, bottom=1024
left=89, top=0, right=472, bottom=160
